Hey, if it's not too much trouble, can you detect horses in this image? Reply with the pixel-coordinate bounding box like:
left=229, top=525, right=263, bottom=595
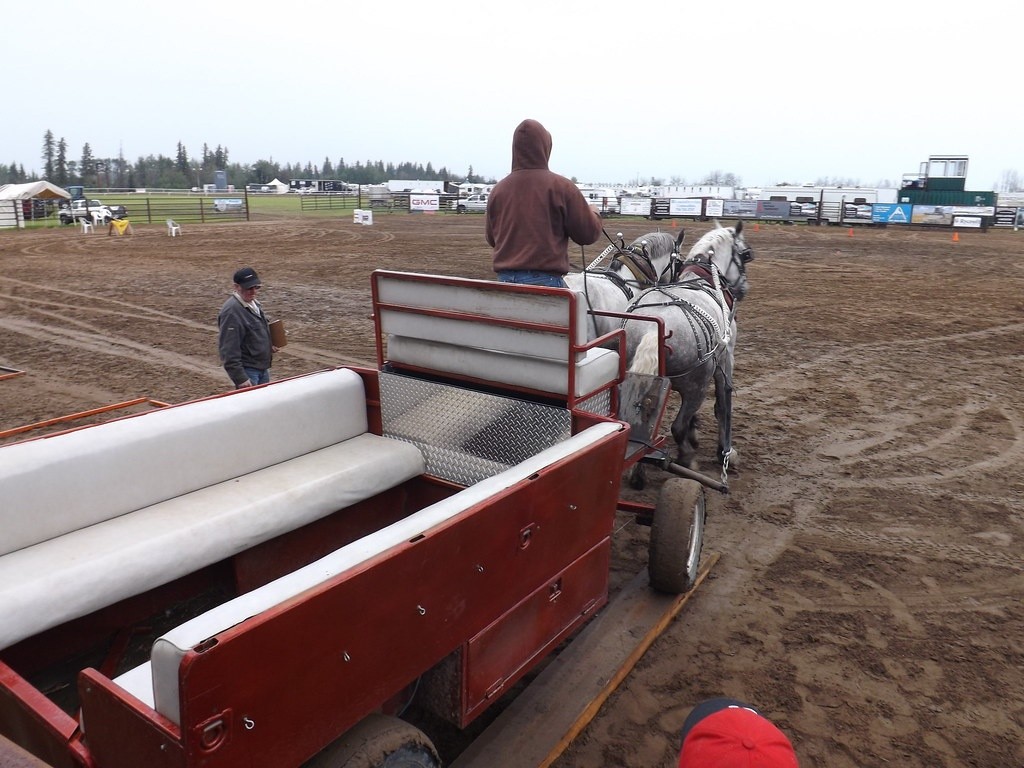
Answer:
left=562, top=227, right=685, bottom=348
left=618, top=219, right=750, bottom=492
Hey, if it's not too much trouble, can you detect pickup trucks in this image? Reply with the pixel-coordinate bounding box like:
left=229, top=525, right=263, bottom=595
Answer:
left=59, top=199, right=127, bottom=224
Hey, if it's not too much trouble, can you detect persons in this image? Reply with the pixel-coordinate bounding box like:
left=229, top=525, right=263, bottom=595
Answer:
left=218, top=267, right=280, bottom=388
left=676, top=699, right=800, bottom=768
left=486, top=119, right=603, bottom=287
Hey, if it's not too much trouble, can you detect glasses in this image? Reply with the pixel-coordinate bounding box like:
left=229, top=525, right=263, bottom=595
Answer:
left=248, top=286, right=261, bottom=290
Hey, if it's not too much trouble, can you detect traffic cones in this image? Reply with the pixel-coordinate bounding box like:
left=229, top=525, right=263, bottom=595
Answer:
left=952, top=231, right=959, bottom=242
left=849, top=227, right=853, bottom=237
left=671, top=218, right=676, bottom=229
left=754, top=221, right=760, bottom=232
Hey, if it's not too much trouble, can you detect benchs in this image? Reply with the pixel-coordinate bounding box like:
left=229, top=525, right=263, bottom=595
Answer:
left=0, top=366, right=426, bottom=653
left=368, top=268, right=626, bottom=401
left=80, top=421, right=624, bottom=734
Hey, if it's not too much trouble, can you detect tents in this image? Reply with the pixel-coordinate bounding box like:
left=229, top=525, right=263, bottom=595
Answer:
left=0, top=181, right=77, bottom=226
left=261, top=178, right=289, bottom=193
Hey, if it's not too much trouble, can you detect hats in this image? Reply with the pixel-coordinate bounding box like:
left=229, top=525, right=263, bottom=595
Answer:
left=233, top=268, right=264, bottom=290
left=679, top=698, right=799, bottom=767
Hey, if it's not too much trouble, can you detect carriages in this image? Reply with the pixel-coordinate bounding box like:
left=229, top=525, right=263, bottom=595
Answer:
left=0, top=213, right=757, bottom=768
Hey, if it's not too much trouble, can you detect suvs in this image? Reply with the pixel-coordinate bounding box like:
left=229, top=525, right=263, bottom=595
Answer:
left=451, top=195, right=489, bottom=213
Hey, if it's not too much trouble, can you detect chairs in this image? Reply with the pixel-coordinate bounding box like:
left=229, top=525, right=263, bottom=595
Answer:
left=78, top=216, right=94, bottom=234
left=166, top=218, right=182, bottom=237
